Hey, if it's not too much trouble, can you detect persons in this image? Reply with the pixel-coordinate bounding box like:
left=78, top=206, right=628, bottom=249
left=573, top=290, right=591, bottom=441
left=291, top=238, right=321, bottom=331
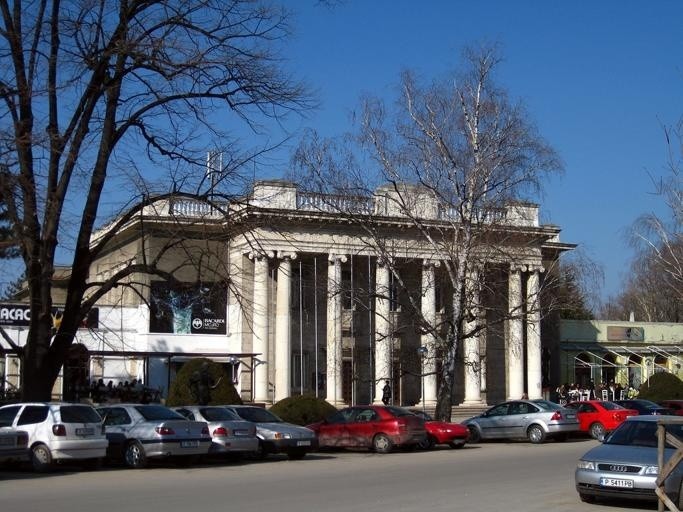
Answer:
left=556, top=383, right=640, bottom=400
left=382, top=380, right=392, bottom=405
left=92, top=378, right=144, bottom=392
left=194, top=319, right=201, bottom=327
left=520, top=393, right=529, bottom=408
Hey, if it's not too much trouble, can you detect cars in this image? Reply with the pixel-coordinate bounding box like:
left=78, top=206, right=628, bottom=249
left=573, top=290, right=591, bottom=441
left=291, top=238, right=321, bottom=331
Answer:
left=577, top=414, right=683, bottom=511
left=462, top=394, right=682, bottom=442
